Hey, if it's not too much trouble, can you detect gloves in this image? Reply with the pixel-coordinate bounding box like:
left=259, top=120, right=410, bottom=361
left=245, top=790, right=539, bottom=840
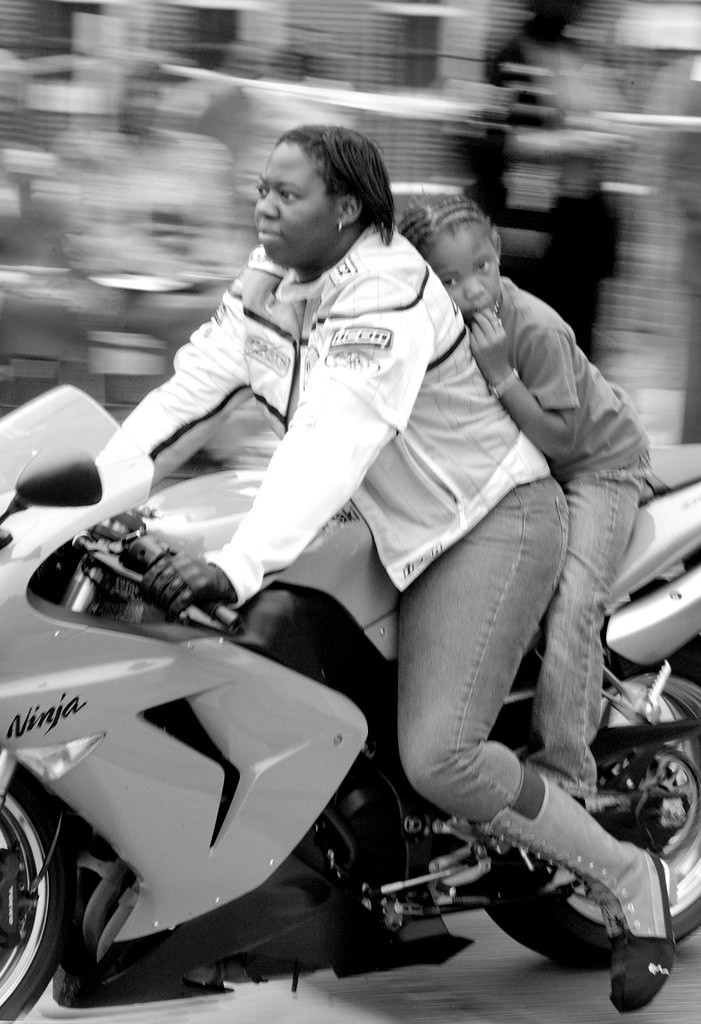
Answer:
left=139, top=555, right=238, bottom=624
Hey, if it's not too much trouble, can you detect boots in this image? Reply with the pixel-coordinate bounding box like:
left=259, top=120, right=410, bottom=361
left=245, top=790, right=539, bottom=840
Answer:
left=477, top=774, right=677, bottom=1012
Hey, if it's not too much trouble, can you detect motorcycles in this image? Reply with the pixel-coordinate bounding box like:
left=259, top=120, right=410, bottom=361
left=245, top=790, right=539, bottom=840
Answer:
left=0, top=381, right=701, bottom=1024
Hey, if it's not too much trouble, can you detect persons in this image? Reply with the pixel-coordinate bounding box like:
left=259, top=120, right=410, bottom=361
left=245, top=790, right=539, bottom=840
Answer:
left=397, top=193, right=651, bottom=798
left=121, top=124, right=676, bottom=1013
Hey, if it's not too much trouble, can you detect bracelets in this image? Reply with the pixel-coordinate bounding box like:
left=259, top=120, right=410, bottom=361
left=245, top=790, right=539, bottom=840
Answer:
left=488, top=367, right=520, bottom=398
